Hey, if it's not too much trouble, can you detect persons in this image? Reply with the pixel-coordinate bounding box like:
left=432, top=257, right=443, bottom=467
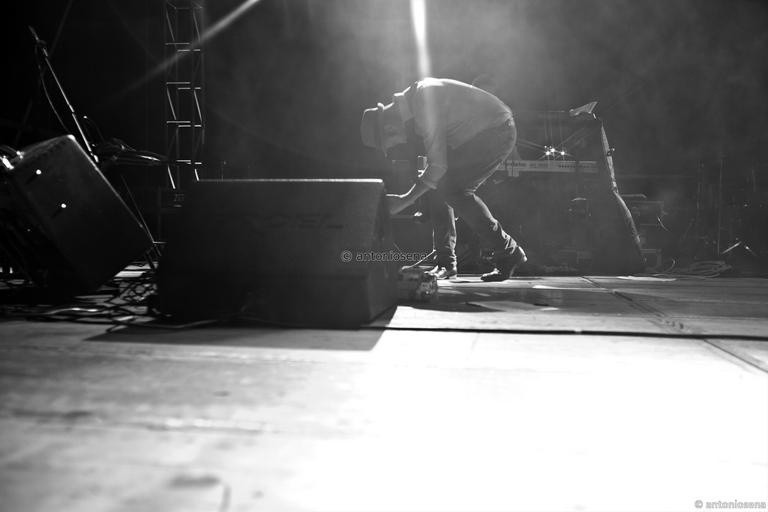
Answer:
left=361, top=76, right=527, bottom=282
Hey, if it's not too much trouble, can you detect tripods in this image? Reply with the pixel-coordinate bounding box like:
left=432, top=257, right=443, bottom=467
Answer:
left=676, top=151, right=768, bottom=267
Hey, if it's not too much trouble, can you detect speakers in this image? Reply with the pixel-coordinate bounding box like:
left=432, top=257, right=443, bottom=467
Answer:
left=156, top=177, right=397, bottom=327
left=0, top=135, right=153, bottom=301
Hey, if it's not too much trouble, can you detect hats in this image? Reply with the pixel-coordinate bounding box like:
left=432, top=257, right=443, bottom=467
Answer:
left=359, top=101, right=389, bottom=159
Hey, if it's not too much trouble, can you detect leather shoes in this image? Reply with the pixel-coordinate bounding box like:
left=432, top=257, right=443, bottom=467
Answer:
left=480, top=247, right=529, bottom=282
left=424, top=263, right=460, bottom=283
